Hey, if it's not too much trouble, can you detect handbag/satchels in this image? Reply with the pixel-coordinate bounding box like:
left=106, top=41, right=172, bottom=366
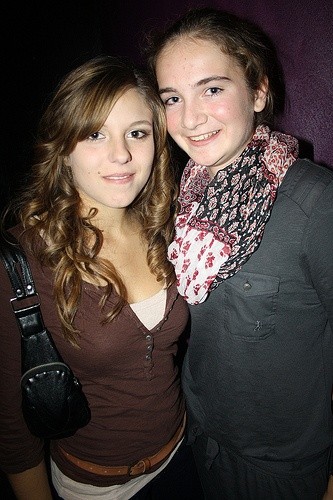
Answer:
left=0, top=231, right=90, bottom=441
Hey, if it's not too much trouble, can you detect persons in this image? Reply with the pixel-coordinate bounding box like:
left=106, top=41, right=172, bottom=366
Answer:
left=0, top=55, right=202, bottom=500
left=138, top=6, right=332, bottom=500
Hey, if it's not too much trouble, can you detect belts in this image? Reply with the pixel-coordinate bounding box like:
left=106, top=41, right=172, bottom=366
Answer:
left=52, top=415, right=188, bottom=476
